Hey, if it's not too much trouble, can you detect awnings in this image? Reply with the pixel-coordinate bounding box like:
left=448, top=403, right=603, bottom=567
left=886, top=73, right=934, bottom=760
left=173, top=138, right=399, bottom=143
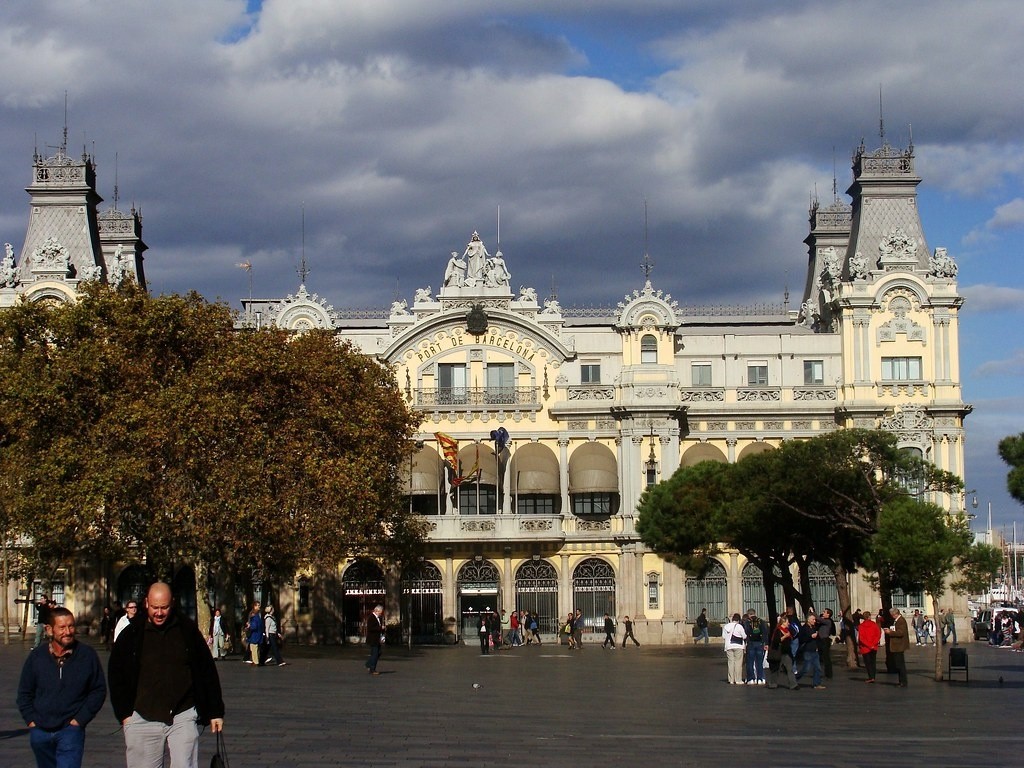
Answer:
left=398, top=442, right=443, bottom=496
left=455, top=443, right=619, bottom=495
left=681, top=442, right=774, bottom=468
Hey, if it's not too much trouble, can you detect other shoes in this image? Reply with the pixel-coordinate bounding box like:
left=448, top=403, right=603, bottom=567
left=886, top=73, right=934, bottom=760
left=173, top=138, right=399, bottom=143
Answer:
left=278, top=662, right=286, bottom=666
left=916, top=641, right=959, bottom=646
left=243, top=660, right=253, bottom=664
left=264, top=658, right=273, bottom=665
left=481, top=639, right=640, bottom=654
left=758, top=679, right=765, bottom=685
left=865, top=679, right=875, bottom=683
left=746, top=679, right=757, bottom=685
left=729, top=682, right=734, bottom=685
left=368, top=671, right=380, bottom=675
left=896, top=683, right=908, bottom=689
left=736, top=681, right=744, bottom=685
left=791, top=686, right=800, bottom=690
left=813, top=685, right=826, bottom=690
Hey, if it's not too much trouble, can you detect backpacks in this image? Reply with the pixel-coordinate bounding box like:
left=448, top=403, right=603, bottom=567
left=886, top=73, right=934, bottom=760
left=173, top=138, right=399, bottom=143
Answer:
left=747, top=618, right=763, bottom=641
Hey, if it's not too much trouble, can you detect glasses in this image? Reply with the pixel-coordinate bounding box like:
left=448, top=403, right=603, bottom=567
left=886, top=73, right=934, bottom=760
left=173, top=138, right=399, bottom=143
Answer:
left=127, top=606, right=138, bottom=609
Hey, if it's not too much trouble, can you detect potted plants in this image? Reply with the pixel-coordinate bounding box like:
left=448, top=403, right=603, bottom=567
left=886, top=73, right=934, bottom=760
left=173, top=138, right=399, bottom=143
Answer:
left=444, top=630, right=456, bottom=644
left=443, top=616, right=456, bottom=625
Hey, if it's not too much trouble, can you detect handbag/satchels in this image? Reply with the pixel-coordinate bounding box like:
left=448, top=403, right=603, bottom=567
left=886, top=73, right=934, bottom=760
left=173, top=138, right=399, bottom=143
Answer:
left=565, top=624, right=571, bottom=633
left=223, top=637, right=233, bottom=649
left=211, top=726, right=229, bottom=768
left=730, top=624, right=744, bottom=646
left=763, top=649, right=770, bottom=669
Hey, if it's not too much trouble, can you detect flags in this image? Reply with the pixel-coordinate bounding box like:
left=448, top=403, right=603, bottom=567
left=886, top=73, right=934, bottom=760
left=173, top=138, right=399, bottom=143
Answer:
left=433, top=432, right=477, bottom=488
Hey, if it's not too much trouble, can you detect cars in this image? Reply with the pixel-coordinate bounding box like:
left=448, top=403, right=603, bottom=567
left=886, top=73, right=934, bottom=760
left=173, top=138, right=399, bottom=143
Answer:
left=968, top=589, right=1024, bottom=619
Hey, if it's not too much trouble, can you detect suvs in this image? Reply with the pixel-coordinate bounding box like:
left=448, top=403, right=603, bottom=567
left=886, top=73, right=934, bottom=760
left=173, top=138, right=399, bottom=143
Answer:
left=973, top=606, right=1020, bottom=642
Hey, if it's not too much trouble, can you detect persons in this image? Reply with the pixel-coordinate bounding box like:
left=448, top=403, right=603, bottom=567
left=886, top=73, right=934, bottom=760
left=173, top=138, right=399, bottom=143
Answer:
left=461, top=231, right=491, bottom=287
left=16, top=608, right=108, bottom=768
left=565, top=609, right=584, bottom=650
left=365, top=604, right=387, bottom=675
left=108, top=582, right=225, bottom=768
left=242, top=601, right=287, bottom=666
left=693, top=608, right=709, bottom=646
left=994, top=608, right=1024, bottom=653
left=602, top=613, right=615, bottom=650
left=102, top=599, right=138, bottom=652
left=486, top=250, right=512, bottom=287
left=722, top=606, right=957, bottom=690
left=32, top=595, right=60, bottom=651
left=476, top=609, right=542, bottom=655
left=209, top=609, right=229, bottom=662
left=620, top=615, right=641, bottom=649
left=444, top=251, right=467, bottom=288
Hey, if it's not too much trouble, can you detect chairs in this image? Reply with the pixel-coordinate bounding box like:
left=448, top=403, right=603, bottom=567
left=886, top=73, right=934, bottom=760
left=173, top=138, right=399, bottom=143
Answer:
left=949, top=647, right=969, bottom=684
